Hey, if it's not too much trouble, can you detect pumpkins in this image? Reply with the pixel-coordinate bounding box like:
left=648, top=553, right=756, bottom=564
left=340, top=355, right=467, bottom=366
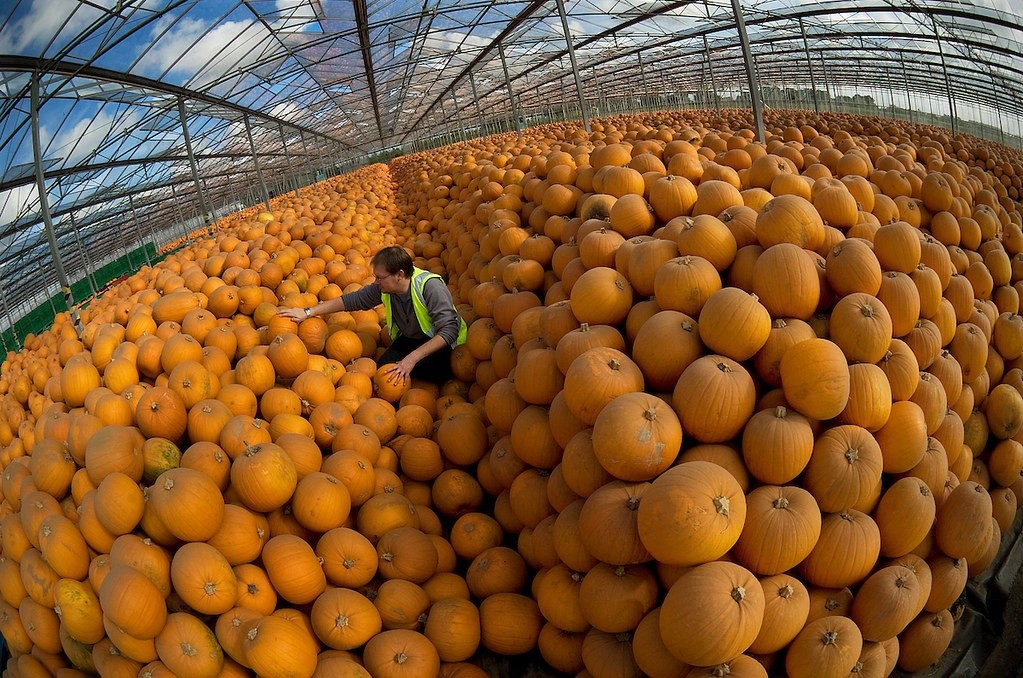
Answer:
left=0, top=108, right=1023, bottom=678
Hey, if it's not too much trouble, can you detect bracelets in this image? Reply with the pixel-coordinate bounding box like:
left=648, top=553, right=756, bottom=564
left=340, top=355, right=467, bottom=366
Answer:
left=304, top=307, right=310, bottom=319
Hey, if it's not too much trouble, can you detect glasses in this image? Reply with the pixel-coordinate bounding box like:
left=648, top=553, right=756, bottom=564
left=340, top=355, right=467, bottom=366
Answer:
left=372, top=272, right=398, bottom=280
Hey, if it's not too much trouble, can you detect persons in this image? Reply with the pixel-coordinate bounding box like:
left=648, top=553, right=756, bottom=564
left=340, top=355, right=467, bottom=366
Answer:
left=276, top=247, right=467, bottom=386
